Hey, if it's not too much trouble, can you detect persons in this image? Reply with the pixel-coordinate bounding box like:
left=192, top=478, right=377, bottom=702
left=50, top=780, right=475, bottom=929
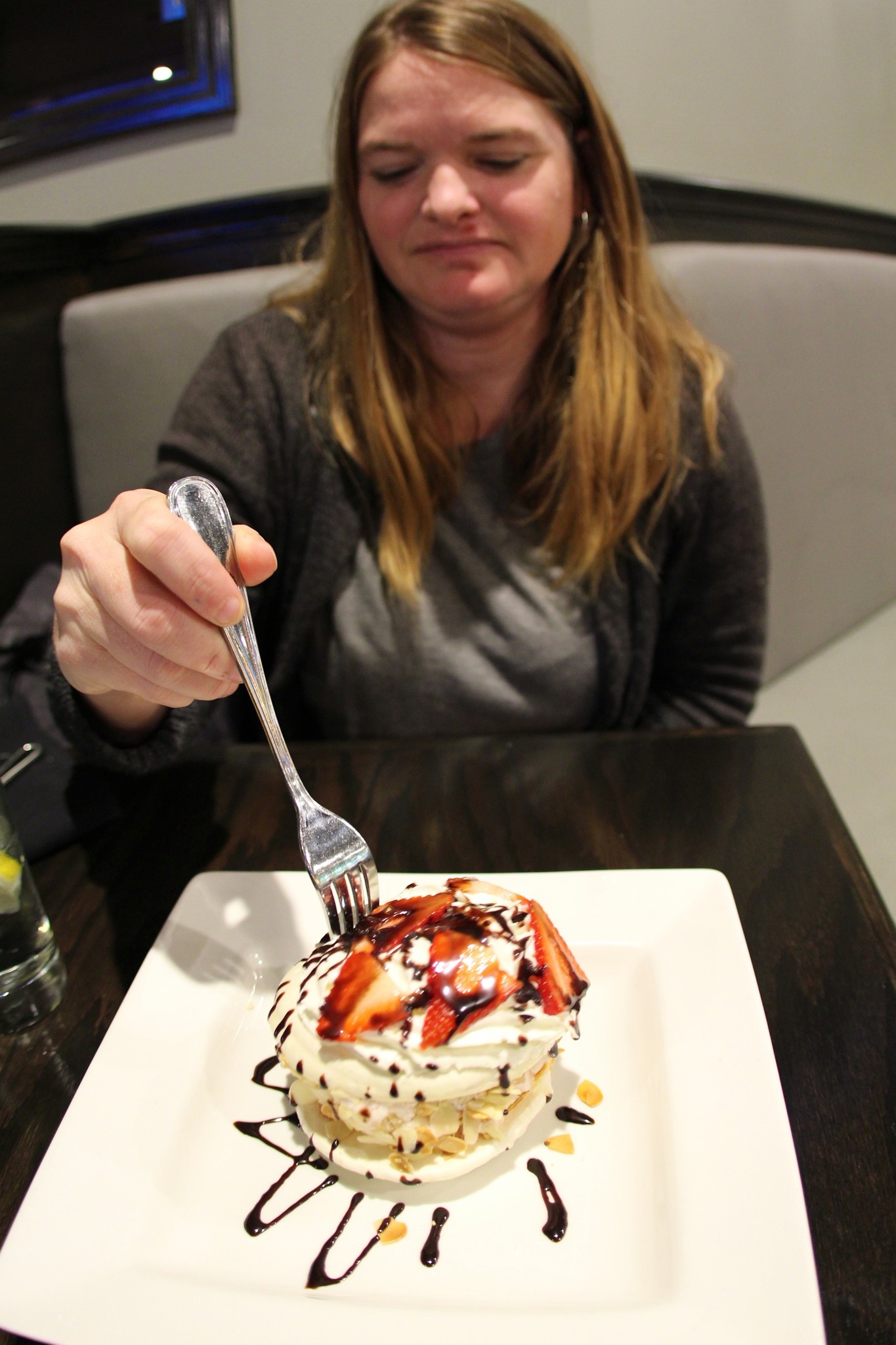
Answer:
left=0, top=0, right=769, bottom=774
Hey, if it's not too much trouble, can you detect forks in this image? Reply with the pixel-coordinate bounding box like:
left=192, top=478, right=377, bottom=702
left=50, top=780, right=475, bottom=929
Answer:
left=167, top=476, right=382, bottom=936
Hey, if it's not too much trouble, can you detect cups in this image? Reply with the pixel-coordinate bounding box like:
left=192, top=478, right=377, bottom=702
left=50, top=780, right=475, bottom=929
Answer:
left=0, top=811, right=68, bottom=1034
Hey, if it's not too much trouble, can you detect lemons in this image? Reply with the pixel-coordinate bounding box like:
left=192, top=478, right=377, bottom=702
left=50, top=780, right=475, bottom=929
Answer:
left=0, top=850, right=24, bottom=914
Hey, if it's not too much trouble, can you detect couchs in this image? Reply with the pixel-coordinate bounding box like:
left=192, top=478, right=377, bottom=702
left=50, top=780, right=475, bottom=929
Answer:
left=65, top=239, right=896, bottom=928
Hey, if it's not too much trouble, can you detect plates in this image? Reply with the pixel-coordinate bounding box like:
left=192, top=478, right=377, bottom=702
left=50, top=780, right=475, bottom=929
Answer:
left=0, top=868, right=829, bottom=1344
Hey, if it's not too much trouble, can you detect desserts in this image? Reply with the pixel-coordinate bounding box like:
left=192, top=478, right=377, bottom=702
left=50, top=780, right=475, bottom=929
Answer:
left=266, top=873, right=589, bottom=1185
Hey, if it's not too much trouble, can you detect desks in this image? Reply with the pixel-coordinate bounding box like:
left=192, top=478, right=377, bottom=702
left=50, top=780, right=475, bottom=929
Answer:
left=0, top=729, right=896, bottom=1345
